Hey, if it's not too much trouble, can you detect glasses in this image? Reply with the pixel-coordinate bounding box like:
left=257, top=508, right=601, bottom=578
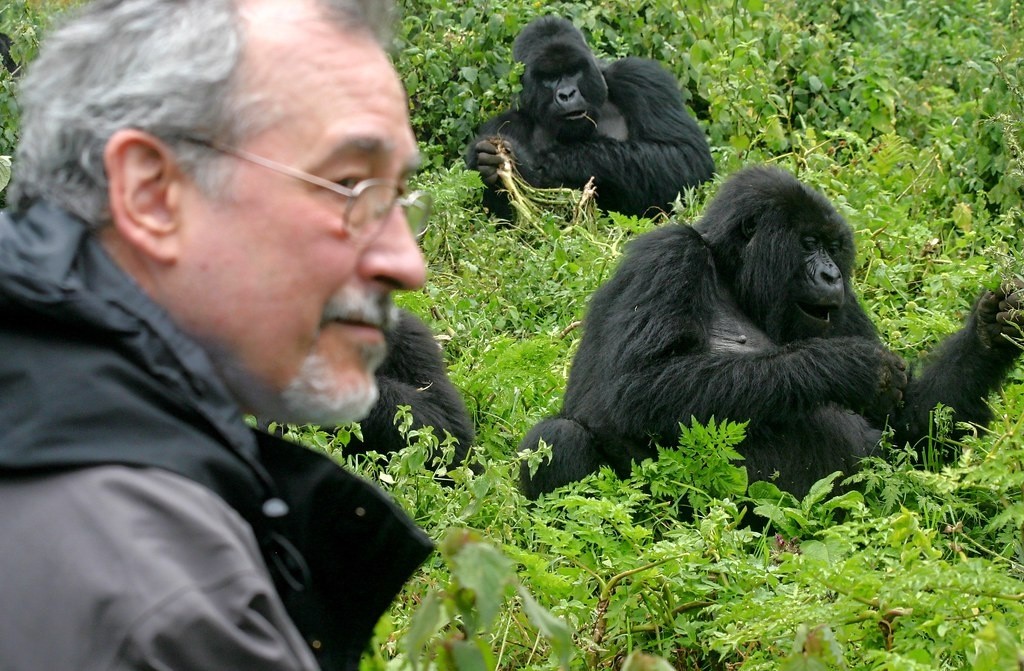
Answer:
left=169, top=130, right=432, bottom=243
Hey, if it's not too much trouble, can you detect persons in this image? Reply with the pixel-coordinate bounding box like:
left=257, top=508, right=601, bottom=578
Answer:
left=0, top=0, right=437, bottom=670
